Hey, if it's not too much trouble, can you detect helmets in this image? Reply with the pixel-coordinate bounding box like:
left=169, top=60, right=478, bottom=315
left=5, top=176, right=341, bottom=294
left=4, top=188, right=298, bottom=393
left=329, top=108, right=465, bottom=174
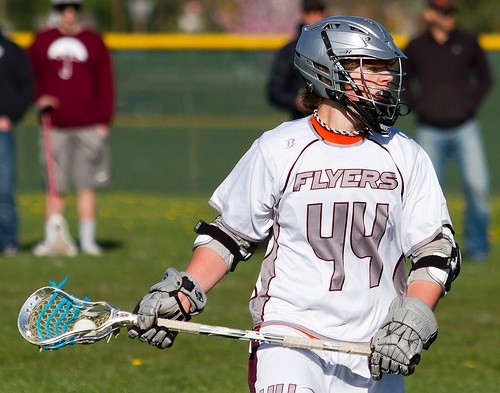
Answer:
left=292, top=15, right=410, bottom=137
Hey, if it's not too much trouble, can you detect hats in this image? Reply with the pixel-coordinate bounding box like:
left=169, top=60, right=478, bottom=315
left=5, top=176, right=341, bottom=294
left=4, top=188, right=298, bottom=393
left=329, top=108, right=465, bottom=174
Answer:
left=301, top=0, right=324, bottom=14
left=51, top=0, right=84, bottom=14
left=426, top=0, right=459, bottom=15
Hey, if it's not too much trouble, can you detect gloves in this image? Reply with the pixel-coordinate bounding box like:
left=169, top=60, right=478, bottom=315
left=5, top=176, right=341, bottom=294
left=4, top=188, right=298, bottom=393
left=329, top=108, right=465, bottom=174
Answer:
left=127, top=267, right=207, bottom=349
left=368, top=296, right=439, bottom=381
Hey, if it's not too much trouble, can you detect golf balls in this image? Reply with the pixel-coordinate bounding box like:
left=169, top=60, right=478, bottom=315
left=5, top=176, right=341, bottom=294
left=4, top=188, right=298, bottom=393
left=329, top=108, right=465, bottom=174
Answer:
left=34, top=116, right=78, bottom=255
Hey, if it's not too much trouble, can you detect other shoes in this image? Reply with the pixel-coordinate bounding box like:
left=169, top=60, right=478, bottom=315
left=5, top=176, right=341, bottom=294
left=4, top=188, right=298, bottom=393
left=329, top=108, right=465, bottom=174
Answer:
left=5, top=245, right=17, bottom=254
left=81, top=243, right=103, bottom=258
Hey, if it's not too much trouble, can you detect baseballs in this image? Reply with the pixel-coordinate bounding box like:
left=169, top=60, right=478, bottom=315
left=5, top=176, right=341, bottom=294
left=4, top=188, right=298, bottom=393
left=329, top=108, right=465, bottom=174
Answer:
left=73, top=315, right=96, bottom=331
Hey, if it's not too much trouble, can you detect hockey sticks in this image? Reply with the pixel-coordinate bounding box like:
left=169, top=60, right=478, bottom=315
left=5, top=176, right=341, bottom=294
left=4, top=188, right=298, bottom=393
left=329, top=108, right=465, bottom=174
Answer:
left=15, top=283, right=372, bottom=352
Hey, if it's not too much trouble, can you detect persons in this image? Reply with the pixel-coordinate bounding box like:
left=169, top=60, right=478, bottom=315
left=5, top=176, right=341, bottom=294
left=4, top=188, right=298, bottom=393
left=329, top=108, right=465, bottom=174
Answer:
left=0, top=0, right=494, bottom=261
left=125, top=17, right=461, bottom=393
